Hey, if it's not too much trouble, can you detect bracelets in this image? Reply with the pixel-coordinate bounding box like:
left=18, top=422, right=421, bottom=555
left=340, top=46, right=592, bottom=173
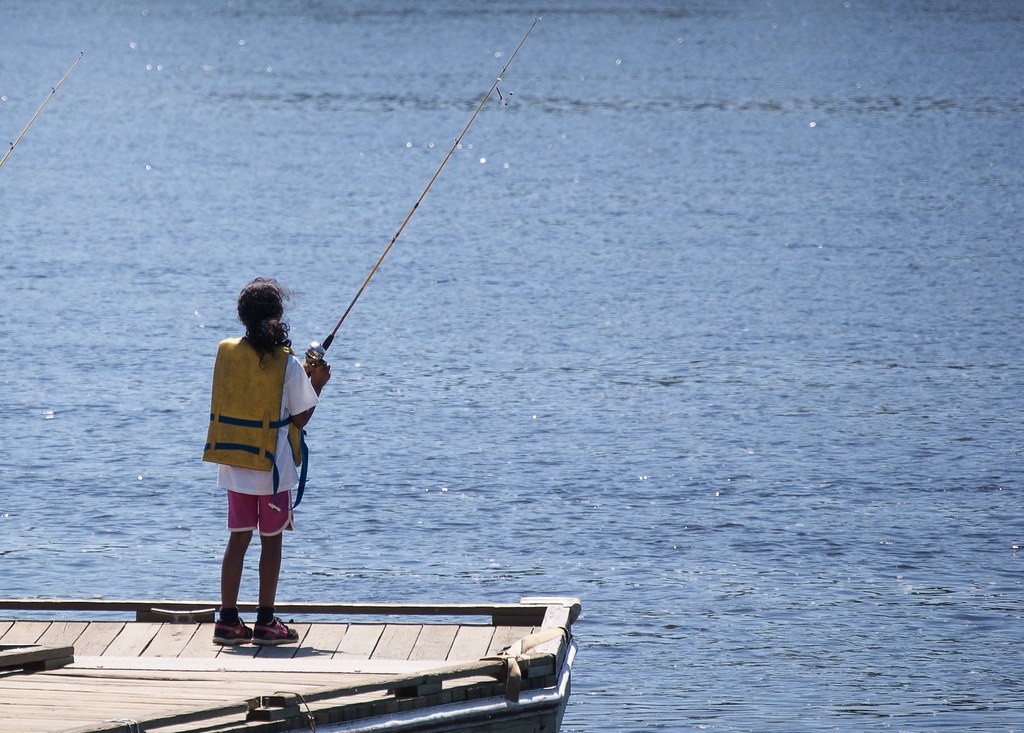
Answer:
left=312, top=382, right=323, bottom=389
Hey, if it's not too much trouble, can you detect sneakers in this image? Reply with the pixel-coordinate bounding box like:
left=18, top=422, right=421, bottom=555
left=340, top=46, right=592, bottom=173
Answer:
left=212, top=619, right=252, bottom=645
left=251, top=620, right=298, bottom=644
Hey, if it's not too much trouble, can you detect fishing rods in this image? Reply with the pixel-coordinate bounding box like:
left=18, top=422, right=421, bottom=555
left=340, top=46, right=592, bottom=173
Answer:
left=299, top=10, right=542, bottom=376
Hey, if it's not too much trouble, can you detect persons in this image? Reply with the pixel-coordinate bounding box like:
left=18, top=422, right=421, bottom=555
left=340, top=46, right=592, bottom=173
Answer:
left=199, top=277, right=333, bottom=645
